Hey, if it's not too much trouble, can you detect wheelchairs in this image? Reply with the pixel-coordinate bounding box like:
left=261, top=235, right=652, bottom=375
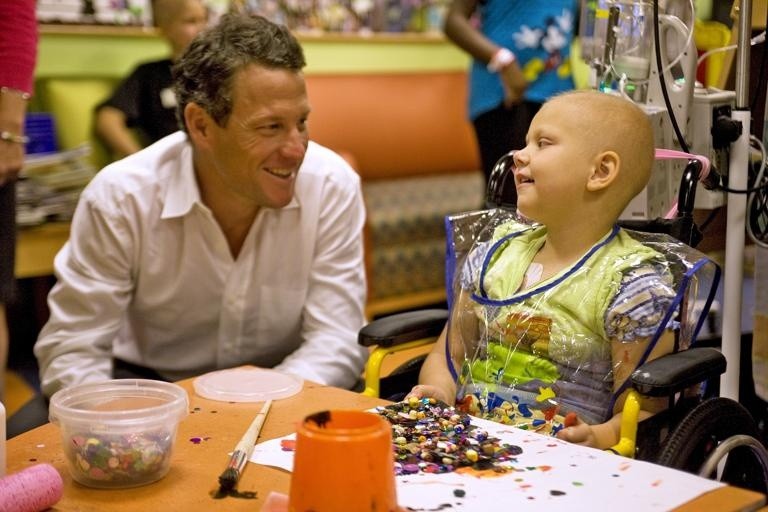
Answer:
left=356, top=148, right=768, bottom=492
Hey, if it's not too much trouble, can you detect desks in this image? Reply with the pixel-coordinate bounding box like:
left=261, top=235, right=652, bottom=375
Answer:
left=2, top=363, right=766, bottom=510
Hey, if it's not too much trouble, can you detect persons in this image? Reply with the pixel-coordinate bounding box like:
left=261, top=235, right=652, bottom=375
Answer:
left=91, top=0, right=216, bottom=160
left=442, top=1, right=581, bottom=211
left=395, top=86, right=677, bottom=451
left=30, top=16, right=368, bottom=403
left=0, top=0, right=51, bottom=438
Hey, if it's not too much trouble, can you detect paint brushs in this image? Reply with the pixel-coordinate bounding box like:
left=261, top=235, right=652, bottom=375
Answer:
left=218, top=399, right=272, bottom=488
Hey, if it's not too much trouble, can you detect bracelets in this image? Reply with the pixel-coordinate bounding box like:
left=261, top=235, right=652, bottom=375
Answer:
left=485, top=45, right=516, bottom=76
left=0, top=129, right=32, bottom=145
left=590, top=421, right=618, bottom=449
